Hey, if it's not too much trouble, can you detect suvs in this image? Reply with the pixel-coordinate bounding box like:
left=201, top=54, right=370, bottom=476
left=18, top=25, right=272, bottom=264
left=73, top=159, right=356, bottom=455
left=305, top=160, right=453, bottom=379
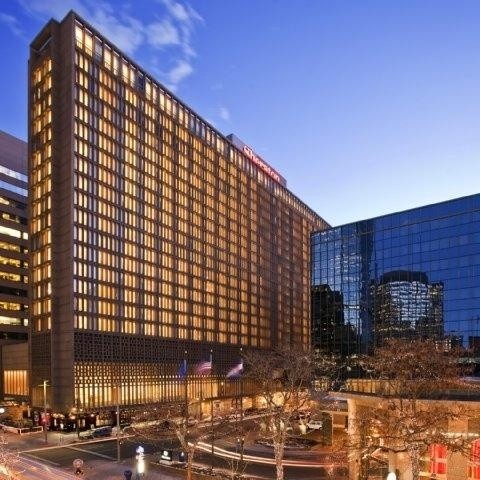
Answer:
left=306, top=419, right=324, bottom=431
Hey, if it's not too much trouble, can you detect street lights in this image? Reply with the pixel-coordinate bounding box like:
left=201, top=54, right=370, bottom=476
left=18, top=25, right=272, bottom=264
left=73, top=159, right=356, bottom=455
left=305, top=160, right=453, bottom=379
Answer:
left=43, top=379, right=49, bottom=428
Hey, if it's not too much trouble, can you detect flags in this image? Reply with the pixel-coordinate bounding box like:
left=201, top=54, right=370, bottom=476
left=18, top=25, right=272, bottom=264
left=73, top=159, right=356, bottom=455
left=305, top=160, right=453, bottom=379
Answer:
left=193, top=355, right=213, bottom=375
left=225, top=357, right=244, bottom=380
left=177, top=357, right=188, bottom=382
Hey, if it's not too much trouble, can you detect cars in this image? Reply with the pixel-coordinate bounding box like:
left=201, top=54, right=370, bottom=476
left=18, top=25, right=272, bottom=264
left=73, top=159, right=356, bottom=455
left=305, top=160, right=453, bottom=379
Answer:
left=82, top=410, right=245, bottom=439
